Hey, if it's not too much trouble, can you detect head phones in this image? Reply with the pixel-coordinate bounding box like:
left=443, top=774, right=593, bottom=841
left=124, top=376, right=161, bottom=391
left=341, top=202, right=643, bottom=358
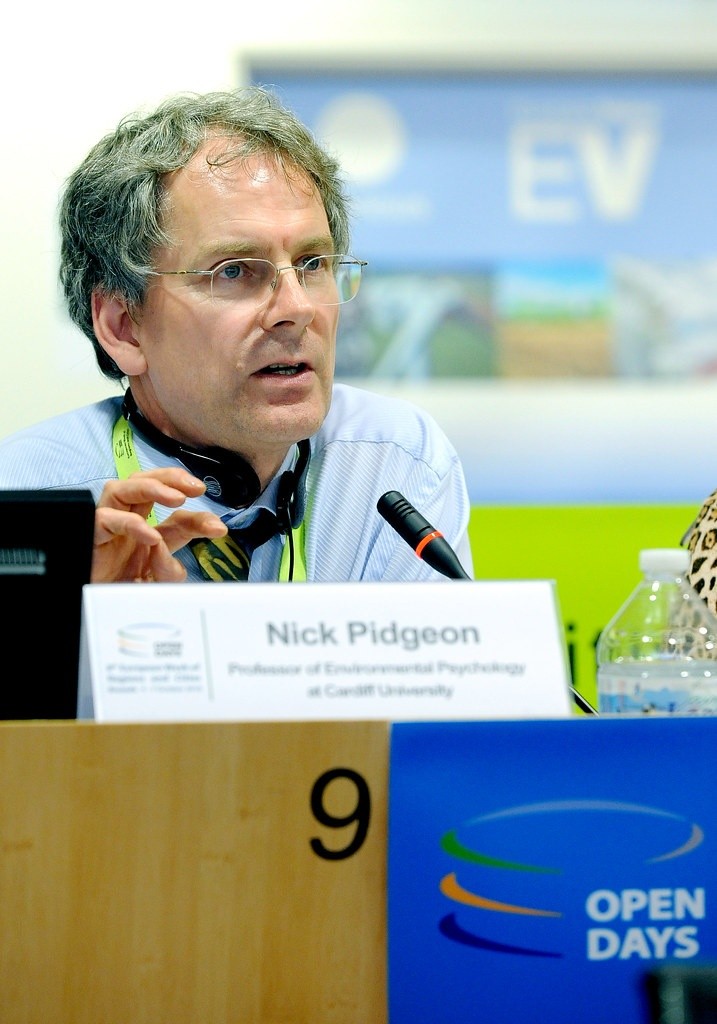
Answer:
left=120, top=385, right=313, bottom=528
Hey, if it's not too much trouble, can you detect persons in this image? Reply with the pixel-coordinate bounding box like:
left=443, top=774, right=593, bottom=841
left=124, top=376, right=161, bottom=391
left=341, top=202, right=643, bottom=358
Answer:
left=0, top=82, right=475, bottom=599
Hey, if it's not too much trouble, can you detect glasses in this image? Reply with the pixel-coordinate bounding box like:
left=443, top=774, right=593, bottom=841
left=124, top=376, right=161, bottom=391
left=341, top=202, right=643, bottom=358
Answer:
left=144, top=254, right=367, bottom=308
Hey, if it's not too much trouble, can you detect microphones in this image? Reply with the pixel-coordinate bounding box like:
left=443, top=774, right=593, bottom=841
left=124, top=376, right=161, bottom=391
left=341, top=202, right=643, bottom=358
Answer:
left=378, top=490, right=598, bottom=714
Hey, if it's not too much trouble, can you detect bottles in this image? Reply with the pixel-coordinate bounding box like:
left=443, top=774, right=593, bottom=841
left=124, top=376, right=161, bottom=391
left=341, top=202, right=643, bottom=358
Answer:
left=596, top=546, right=716, bottom=718
